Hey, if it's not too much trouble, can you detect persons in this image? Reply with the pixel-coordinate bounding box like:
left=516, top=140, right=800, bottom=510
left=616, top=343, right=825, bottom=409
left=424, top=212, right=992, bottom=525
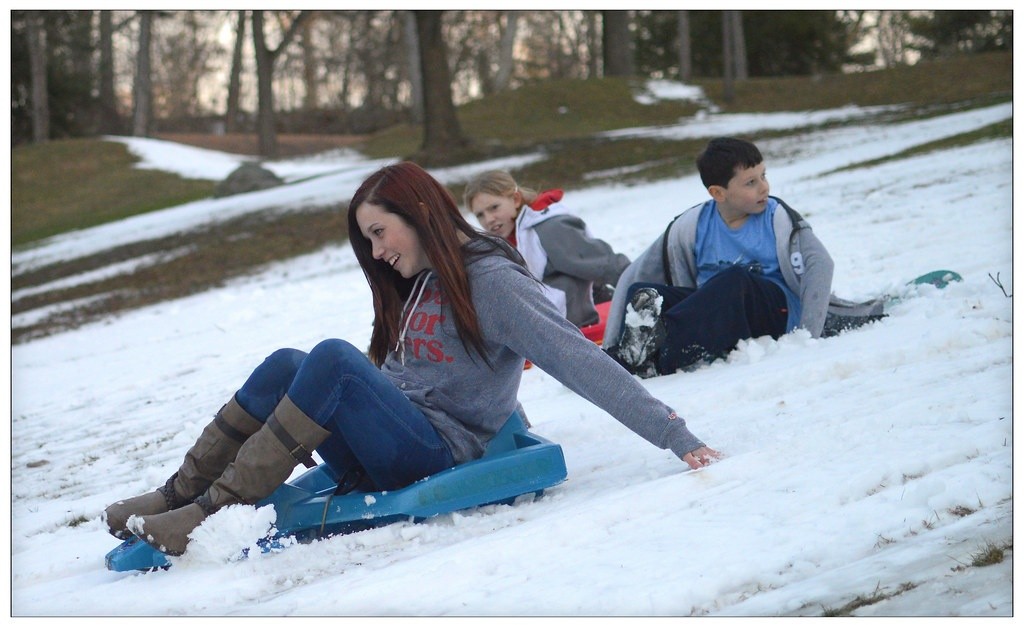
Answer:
left=463, top=167, right=632, bottom=370
left=100, top=159, right=721, bottom=557
left=601, top=136, right=889, bottom=379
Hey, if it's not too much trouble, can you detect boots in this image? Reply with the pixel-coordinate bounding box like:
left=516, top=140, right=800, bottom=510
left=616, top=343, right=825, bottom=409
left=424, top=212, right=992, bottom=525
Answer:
left=100, top=395, right=264, bottom=542
left=127, top=392, right=332, bottom=557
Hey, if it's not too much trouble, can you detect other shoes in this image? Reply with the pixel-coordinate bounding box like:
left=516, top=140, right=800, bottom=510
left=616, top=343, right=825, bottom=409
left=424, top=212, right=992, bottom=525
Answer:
left=616, top=287, right=665, bottom=370
